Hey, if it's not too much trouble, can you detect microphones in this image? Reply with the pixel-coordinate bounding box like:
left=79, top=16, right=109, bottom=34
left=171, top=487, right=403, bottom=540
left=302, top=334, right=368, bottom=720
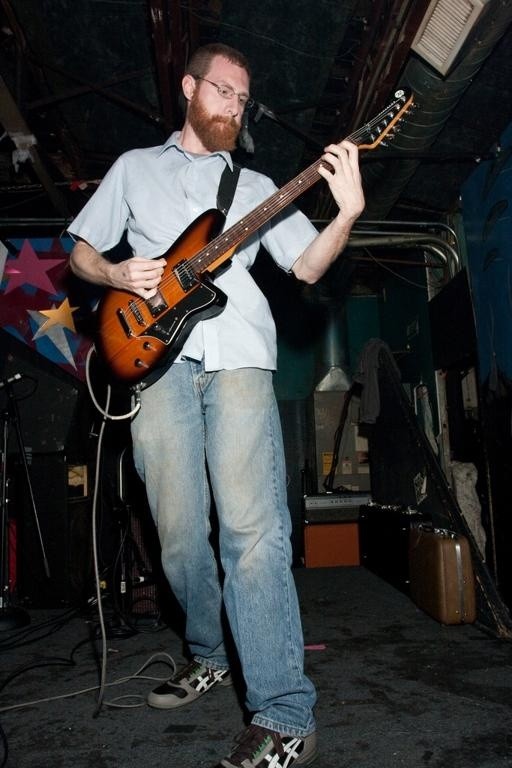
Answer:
left=0, top=373, right=22, bottom=388
left=247, top=96, right=268, bottom=123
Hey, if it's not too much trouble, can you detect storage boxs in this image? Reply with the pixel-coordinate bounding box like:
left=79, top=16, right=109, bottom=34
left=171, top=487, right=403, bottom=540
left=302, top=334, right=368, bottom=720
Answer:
left=300, top=500, right=364, bottom=570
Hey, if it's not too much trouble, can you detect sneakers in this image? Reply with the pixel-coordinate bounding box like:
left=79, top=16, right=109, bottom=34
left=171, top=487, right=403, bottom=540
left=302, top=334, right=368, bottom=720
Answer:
left=216, top=724, right=319, bottom=768
left=147, top=660, right=231, bottom=710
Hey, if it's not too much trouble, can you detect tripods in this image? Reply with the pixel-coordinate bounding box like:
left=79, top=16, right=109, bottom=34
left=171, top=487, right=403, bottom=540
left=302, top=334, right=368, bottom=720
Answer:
left=91, top=502, right=162, bottom=636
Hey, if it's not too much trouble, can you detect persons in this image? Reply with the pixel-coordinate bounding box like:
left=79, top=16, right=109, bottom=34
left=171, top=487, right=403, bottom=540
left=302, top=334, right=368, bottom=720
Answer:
left=66, top=43, right=365, bottom=767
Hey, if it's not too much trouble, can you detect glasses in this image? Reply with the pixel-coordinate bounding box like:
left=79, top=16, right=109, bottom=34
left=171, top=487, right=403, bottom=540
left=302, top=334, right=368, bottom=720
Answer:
left=197, top=76, right=254, bottom=108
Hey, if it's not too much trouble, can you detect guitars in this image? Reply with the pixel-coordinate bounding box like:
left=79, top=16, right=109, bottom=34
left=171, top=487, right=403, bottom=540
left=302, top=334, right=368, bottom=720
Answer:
left=93, top=91, right=417, bottom=396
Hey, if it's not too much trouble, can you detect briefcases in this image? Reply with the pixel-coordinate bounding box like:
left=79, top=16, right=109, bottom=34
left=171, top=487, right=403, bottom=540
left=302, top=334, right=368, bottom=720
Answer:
left=409, top=524, right=478, bottom=626
